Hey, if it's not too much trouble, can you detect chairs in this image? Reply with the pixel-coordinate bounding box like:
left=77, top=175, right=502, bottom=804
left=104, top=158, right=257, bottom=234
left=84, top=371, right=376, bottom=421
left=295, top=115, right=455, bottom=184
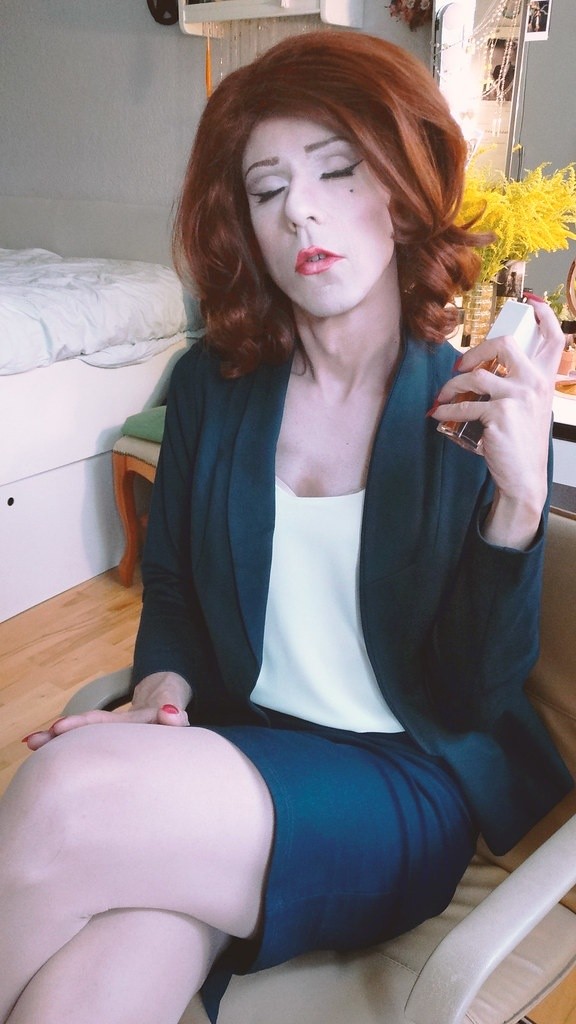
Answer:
left=61, top=515, right=576, bottom=1024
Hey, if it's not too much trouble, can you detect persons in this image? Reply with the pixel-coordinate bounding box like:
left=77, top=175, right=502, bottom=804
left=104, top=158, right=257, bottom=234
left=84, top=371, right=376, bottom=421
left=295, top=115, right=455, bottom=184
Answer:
left=0, top=21, right=557, bottom=1022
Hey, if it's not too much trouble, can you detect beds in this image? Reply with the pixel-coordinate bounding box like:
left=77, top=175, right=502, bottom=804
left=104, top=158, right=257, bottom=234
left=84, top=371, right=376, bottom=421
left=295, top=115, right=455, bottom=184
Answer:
left=1, top=246, right=204, bottom=625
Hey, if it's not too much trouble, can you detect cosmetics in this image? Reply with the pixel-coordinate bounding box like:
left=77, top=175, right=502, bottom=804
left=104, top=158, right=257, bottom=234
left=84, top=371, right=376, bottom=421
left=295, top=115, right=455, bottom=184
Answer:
left=436, top=301, right=544, bottom=455
left=560, top=345, right=575, bottom=375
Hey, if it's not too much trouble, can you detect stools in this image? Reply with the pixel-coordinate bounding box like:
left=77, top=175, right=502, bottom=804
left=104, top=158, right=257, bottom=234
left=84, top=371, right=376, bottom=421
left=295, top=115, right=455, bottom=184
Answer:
left=107, top=405, right=167, bottom=589
